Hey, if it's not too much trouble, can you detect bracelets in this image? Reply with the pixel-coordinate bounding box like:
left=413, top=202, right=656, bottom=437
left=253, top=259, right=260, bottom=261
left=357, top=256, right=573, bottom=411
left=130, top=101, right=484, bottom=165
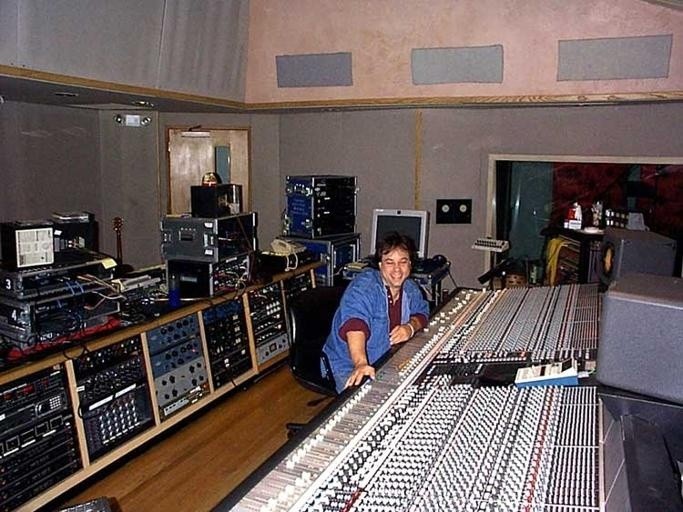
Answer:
left=406, top=321, right=416, bottom=339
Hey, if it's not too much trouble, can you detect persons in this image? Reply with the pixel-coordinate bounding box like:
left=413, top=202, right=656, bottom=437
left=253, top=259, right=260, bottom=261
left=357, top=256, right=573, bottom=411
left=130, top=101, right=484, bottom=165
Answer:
left=199, top=171, right=224, bottom=186
left=315, top=230, right=431, bottom=398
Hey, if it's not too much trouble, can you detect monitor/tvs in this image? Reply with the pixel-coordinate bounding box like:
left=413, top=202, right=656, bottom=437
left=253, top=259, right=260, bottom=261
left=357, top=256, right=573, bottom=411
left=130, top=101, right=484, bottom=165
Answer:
left=369, top=208, right=431, bottom=260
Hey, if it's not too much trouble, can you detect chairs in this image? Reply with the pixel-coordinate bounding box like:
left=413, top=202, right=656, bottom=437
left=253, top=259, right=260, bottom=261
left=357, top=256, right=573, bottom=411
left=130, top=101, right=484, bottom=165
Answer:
left=283, top=285, right=345, bottom=438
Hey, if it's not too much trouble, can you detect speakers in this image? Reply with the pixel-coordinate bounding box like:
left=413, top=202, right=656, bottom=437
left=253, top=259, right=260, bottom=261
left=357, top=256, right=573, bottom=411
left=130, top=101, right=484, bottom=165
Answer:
left=597, top=272, right=683, bottom=405
left=595, top=227, right=678, bottom=285
